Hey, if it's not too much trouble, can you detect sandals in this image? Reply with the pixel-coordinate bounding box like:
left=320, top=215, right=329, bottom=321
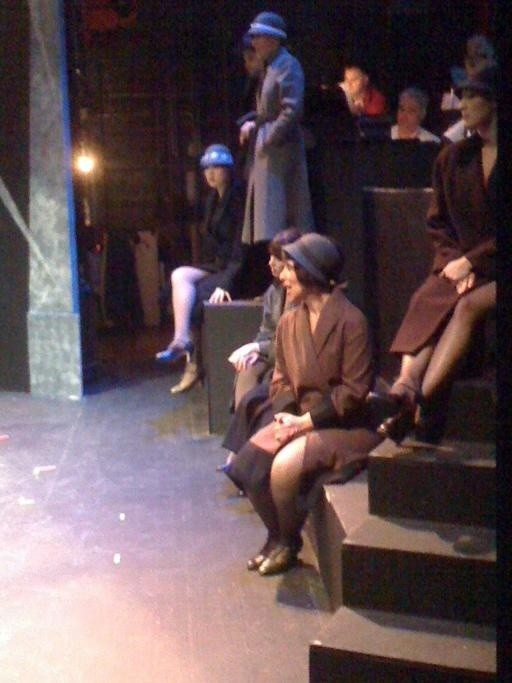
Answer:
left=259, top=541, right=302, bottom=575
left=247, top=542, right=271, bottom=569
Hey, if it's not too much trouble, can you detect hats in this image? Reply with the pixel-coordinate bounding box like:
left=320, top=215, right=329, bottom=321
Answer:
left=281, top=232, right=340, bottom=285
left=247, top=12, right=287, bottom=40
left=453, top=67, right=496, bottom=97
left=199, top=143, right=233, bottom=171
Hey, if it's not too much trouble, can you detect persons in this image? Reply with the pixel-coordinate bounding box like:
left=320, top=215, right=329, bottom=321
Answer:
left=391, top=88, right=440, bottom=142
left=225, top=232, right=383, bottom=576
left=239, top=12, right=315, bottom=246
left=214, top=227, right=302, bottom=474
left=344, top=63, right=385, bottom=115
left=156, top=144, right=253, bottom=395
left=363, top=64, right=512, bottom=445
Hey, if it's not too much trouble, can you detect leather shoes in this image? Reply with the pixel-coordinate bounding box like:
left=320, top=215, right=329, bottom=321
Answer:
left=379, top=415, right=406, bottom=445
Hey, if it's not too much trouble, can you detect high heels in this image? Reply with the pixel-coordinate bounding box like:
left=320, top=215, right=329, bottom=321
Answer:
left=171, top=360, right=204, bottom=394
left=155, top=336, right=194, bottom=364
